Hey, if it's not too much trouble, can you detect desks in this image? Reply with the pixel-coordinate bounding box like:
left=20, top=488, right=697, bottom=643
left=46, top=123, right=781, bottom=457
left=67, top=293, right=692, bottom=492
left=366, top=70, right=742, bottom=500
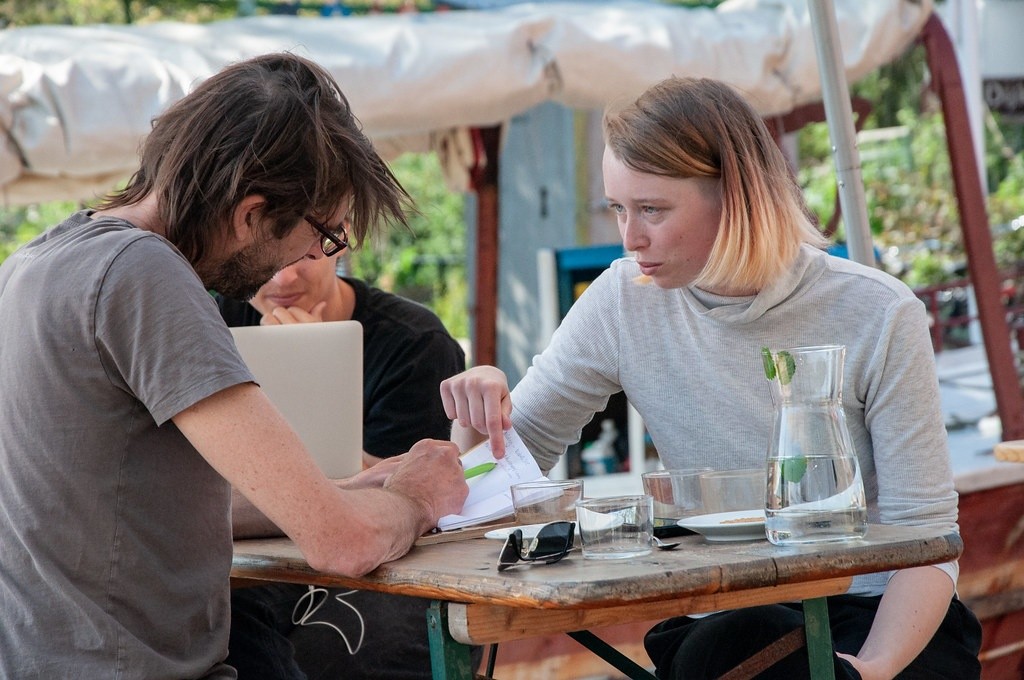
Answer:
left=229, top=501, right=962, bottom=680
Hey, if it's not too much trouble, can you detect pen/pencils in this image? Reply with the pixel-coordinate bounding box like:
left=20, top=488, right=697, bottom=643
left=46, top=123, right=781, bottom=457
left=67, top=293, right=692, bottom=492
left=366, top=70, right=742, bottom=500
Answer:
left=464, top=463, right=498, bottom=479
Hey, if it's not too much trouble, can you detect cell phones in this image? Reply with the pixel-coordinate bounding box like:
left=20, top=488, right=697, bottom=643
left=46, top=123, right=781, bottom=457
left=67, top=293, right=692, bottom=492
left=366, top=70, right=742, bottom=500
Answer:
left=614, top=516, right=701, bottom=538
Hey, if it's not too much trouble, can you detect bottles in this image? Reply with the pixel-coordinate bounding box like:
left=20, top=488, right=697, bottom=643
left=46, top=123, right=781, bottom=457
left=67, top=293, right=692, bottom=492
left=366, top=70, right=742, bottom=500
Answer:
left=763, top=343, right=869, bottom=548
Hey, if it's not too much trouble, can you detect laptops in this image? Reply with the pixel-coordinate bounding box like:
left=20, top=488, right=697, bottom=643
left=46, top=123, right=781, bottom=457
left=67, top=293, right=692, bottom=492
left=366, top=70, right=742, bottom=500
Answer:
left=227, top=320, right=364, bottom=540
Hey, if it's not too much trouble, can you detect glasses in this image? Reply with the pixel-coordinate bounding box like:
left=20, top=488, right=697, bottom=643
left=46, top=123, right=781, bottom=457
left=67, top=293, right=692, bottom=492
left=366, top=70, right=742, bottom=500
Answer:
left=496, top=520, right=577, bottom=571
left=297, top=210, right=350, bottom=257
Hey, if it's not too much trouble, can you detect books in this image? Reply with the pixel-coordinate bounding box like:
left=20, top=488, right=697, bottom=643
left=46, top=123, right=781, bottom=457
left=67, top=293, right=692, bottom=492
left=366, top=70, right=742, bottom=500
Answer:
left=434, top=426, right=564, bottom=533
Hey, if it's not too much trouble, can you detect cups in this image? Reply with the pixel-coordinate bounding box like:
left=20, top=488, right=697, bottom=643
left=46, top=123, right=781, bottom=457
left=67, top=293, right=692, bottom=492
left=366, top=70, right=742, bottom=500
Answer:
left=700, top=467, right=767, bottom=514
left=509, top=479, right=584, bottom=554
left=576, top=494, right=655, bottom=561
left=641, top=468, right=713, bottom=530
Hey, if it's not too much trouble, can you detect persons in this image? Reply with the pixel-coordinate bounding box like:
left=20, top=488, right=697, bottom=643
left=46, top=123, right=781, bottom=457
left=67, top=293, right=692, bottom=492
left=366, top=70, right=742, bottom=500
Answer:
left=1, top=52, right=469, bottom=680
left=214, top=221, right=471, bottom=680
left=437, top=76, right=985, bottom=680
